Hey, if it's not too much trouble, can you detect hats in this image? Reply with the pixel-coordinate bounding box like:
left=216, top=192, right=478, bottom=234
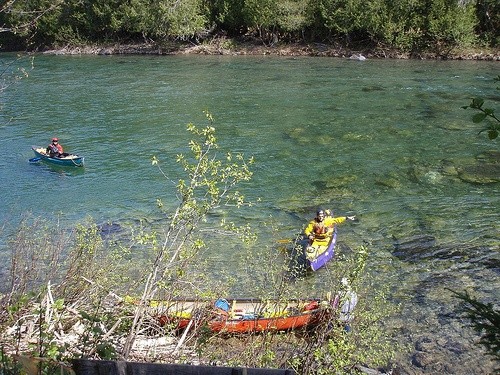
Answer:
left=316, top=209, right=323, bottom=215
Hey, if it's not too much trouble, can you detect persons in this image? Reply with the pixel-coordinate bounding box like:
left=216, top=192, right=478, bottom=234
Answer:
left=328, top=278, right=358, bottom=338
left=305, top=210, right=356, bottom=240
left=46, top=137, right=79, bottom=158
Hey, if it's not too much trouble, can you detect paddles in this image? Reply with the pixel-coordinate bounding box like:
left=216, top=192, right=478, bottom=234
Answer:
left=276, top=237, right=326, bottom=242
left=29, top=153, right=48, bottom=162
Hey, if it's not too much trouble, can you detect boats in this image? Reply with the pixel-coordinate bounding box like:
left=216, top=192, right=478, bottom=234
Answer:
left=305, top=208, right=339, bottom=273
left=31, top=144, right=84, bottom=168
left=115, top=297, right=325, bottom=336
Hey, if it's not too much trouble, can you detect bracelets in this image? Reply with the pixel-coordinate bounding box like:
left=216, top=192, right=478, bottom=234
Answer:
left=346, top=215, right=348, bottom=220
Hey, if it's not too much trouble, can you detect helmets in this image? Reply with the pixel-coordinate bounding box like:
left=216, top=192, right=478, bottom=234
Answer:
left=52, top=138, right=58, bottom=142
